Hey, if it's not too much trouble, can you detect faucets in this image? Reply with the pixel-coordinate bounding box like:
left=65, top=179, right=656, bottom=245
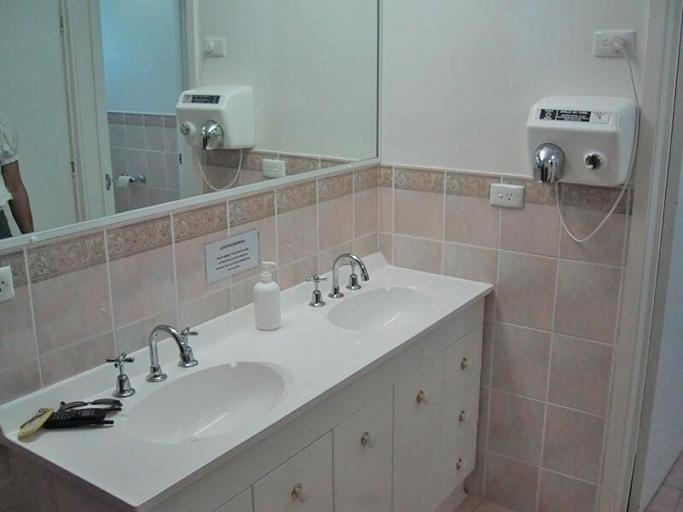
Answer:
left=332, top=253, right=370, bottom=287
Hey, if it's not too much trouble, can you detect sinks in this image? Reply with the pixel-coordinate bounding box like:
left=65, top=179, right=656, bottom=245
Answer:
left=148, top=324, right=198, bottom=368
left=114, top=361, right=296, bottom=444
left=328, top=286, right=436, bottom=332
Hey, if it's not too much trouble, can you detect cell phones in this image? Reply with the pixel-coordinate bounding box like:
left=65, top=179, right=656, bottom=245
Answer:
left=45, top=408, right=113, bottom=430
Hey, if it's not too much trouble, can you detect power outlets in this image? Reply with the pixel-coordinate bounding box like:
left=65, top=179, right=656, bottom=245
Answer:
left=490, top=182, right=525, bottom=209
left=204, top=38, right=228, bottom=57
left=262, top=159, right=286, bottom=178
left=592, top=28, right=638, bottom=58
left=0, top=265, right=15, bottom=301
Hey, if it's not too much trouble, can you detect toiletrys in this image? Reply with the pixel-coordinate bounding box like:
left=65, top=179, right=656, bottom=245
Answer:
left=254, top=262, right=280, bottom=330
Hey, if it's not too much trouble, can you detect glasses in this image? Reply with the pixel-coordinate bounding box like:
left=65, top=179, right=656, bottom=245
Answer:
left=58, top=397, right=124, bottom=414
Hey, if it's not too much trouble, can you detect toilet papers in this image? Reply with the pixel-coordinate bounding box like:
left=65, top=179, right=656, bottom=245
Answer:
left=116, top=175, right=134, bottom=187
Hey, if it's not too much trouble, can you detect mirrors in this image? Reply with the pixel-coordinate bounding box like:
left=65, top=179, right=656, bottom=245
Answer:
left=0, top=1, right=378, bottom=252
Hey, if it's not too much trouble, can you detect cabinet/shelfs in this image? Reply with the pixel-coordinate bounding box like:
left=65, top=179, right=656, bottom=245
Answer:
left=392, top=357, right=451, bottom=512
left=440, top=321, right=483, bottom=482
left=333, top=393, right=392, bottom=508
left=251, top=431, right=331, bottom=512
